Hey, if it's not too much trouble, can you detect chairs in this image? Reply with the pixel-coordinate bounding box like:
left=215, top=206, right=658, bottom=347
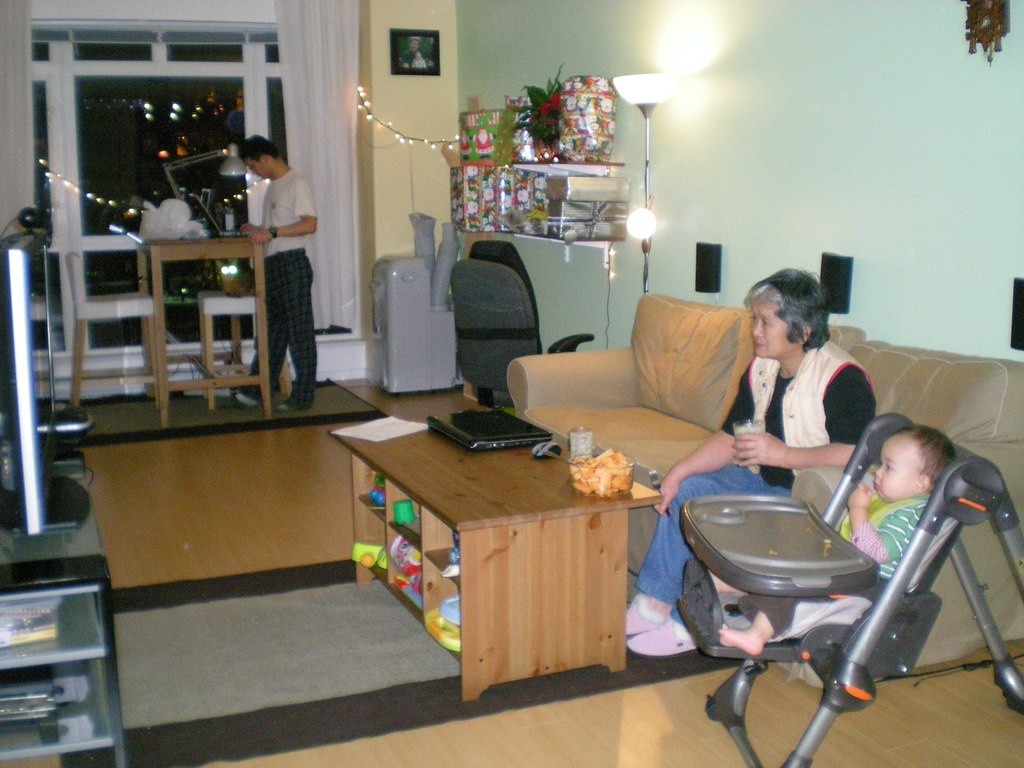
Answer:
left=449, top=239, right=600, bottom=408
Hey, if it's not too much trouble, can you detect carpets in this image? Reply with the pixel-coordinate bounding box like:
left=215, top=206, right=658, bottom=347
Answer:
left=73, top=376, right=389, bottom=447
left=97, top=558, right=739, bottom=765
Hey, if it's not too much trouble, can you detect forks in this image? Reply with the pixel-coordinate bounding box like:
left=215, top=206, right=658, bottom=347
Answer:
left=648, top=471, right=672, bottom=518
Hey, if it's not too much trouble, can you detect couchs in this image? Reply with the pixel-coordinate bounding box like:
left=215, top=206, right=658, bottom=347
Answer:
left=506, top=293, right=1024, bottom=666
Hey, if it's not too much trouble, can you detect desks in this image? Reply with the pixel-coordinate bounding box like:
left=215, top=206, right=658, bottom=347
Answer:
left=133, top=238, right=270, bottom=425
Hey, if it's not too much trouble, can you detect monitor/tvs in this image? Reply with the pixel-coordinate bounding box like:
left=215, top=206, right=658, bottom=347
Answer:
left=0, top=248, right=89, bottom=534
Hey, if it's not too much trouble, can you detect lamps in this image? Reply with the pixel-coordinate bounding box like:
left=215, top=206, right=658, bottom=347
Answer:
left=613, top=70, right=673, bottom=292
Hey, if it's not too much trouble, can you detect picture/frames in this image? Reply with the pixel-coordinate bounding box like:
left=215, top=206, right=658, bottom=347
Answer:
left=389, top=28, right=441, bottom=77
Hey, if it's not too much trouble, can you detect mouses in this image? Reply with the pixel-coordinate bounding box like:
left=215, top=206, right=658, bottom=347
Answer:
left=531, top=442, right=562, bottom=458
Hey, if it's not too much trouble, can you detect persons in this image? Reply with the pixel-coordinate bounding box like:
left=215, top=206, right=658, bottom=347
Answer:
left=627, top=268, right=875, bottom=657
left=219, top=135, right=318, bottom=411
left=708, top=425, right=956, bottom=657
left=402, top=38, right=427, bottom=69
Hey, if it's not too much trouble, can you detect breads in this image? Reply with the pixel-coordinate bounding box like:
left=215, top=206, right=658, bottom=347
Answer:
left=570, top=447, right=633, bottom=497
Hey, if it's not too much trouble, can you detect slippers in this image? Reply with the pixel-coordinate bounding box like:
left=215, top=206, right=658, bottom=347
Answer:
left=627, top=617, right=697, bottom=657
left=217, top=396, right=258, bottom=410
left=274, top=398, right=304, bottom=414
left=625, top=593, right=668, bottom=634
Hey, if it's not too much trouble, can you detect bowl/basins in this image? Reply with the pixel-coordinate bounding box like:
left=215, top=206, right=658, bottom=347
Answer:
left=569, top=455, right=636, bottom=500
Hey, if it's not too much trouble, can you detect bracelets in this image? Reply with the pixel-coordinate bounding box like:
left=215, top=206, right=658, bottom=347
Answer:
left=269, top=227, right=277, bottom=238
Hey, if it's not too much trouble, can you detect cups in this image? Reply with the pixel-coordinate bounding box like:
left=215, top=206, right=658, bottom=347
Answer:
left=733, top=418, right=767, bottom=464
left=568, top=427, right=594, bottom=462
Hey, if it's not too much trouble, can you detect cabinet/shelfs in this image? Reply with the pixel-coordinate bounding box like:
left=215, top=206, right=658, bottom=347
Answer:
left=324, top=423, right=662, bottom=703
left=0, top=461, right=126, bottom=768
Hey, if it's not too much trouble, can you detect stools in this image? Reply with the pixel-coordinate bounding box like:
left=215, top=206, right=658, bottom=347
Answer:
left=64, top=252, right=161, bottom=409
left=195, top=289, right=257, bottom=412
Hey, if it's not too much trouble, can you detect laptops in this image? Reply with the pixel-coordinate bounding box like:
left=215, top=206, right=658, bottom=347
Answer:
left=186, top=193, right=250, bottom=237
left=427, top=411, right=553, bottom=451
